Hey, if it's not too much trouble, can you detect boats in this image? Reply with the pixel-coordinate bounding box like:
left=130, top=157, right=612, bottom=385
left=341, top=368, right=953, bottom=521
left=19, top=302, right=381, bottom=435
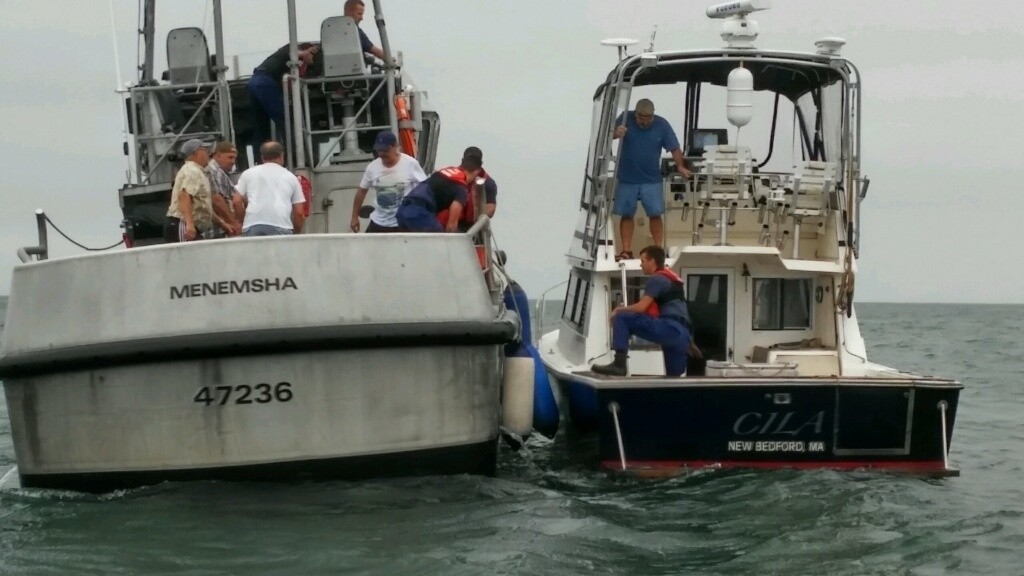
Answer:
left=535, top=0, right=970, bottom=482
left=1, top=0, right=563, bottom=503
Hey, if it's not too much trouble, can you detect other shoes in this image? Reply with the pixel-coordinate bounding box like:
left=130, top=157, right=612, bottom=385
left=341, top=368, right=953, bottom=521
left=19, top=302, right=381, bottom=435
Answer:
left=591, top=362, right=627, bottom=377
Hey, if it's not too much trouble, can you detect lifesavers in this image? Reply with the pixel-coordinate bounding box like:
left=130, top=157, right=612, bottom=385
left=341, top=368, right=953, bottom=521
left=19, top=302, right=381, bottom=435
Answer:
left=396, top=97, right=416, bottom=159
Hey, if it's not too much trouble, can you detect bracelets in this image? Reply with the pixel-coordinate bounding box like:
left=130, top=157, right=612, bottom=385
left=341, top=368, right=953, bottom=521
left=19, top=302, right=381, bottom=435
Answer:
left=217, top=219, right=225, bottom=227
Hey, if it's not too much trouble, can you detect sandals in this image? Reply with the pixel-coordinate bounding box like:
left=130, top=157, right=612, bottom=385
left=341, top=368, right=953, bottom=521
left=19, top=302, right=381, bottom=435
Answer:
left=615, top=250, right=634, bottom=261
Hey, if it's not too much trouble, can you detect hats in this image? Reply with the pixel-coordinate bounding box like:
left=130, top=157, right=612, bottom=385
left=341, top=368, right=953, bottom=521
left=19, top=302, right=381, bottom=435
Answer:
left=180, top=139, right=211, bottom=156
left=214, top=142, right=236, bottom=154
left=374, top=132, right=396, bottom=152
left=636, top=99, right=654, bottom=115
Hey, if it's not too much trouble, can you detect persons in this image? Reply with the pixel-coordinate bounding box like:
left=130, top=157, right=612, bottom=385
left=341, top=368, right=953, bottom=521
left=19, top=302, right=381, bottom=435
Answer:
left=233, top=142, right=306, bottom=237
left=350, top=131, right=426, bottom=233
left=611, top=99, right=691, bottom=261
left=396, top=147, right=498, bottom=269
left=163, top=139, right=240, bottom=243
left=248, top=0, right=396, bottom=134
left=205, top=141, right=246, bottom=239
left=591, top=245, right=691, bottom=378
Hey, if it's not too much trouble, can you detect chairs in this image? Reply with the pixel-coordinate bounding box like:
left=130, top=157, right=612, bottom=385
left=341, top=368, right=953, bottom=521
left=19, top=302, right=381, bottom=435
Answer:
left=166, top=27, right=212, bottom=99
left=700, top=146, right=752, bottom=198
left=786, top=161, right=836, bottom=215
left=321, top=15, right=372, bottom=100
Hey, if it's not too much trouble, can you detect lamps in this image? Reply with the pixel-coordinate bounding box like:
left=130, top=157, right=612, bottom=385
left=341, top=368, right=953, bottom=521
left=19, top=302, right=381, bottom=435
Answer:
left=742, top=263, right=751, bottom=292
